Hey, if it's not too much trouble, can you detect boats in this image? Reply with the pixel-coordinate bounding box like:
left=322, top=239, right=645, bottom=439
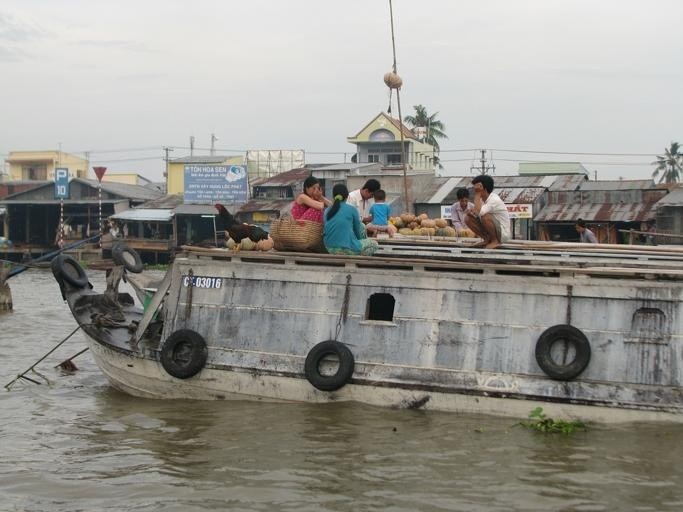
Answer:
left=49, top=231, right=683, bottom=422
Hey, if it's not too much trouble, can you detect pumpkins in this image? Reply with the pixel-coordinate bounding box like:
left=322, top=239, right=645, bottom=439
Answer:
left=226, top=237, right=274, bottom=250
left=388, top=213, right=476, bottom=237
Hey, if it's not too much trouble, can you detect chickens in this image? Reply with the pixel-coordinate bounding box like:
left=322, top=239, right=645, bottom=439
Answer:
left=214, top=203, right=269, bottom=251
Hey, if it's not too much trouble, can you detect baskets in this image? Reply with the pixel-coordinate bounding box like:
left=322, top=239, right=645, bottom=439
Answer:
left=269, top=211, right=324, bottom=252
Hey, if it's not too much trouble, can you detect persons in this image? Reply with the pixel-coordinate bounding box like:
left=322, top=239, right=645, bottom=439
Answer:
left=99, top=220, right=131, bottom=258
left=463, top=174, right=510, bottom=250
left=321, top=184, right=380, bottom=257
left=364, top=190, right=394, bottom=240
left=574, top=218, right=598, bottom=244
left=630, top=218, right=657, bottom=245
left=450, top=188, right=476, bottom=236
left=345, top=178, right=380, bottom=228
left=292, top=176, right=333, bottom=223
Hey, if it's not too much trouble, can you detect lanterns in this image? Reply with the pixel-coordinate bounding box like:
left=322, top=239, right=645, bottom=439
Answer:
left=383, top=72, right=403, bottom=90
